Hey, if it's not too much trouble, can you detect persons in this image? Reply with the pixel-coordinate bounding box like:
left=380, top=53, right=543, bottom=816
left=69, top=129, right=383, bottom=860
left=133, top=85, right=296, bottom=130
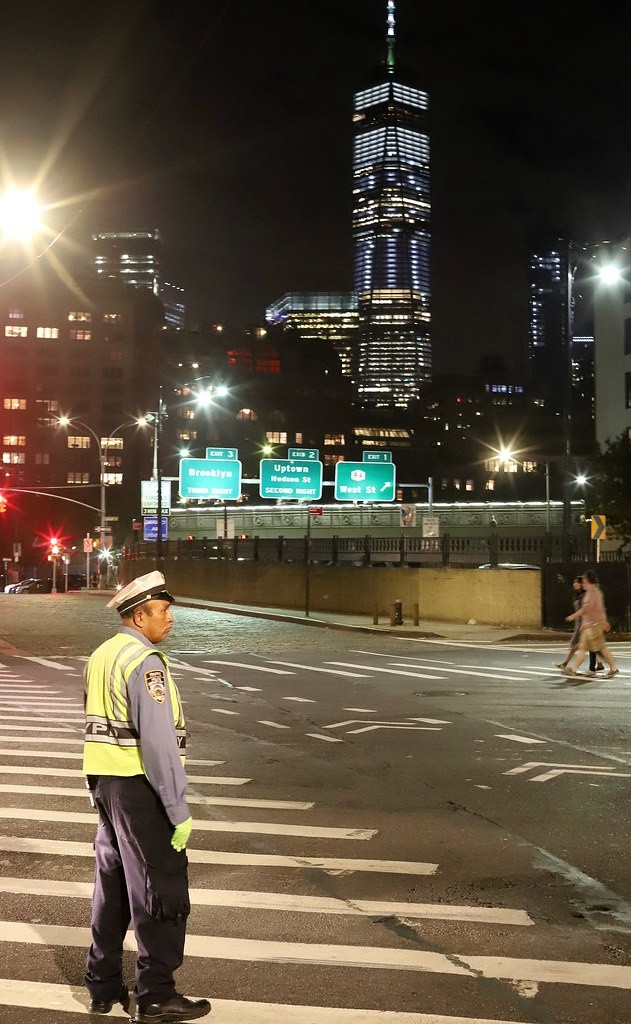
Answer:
left=83, top=570, right=213, bottom=1024
left=552, top=575, right=605, bottom=673
left=561, top=570, right=620, bottom=678
left=89, top=571, right=99, bottom=588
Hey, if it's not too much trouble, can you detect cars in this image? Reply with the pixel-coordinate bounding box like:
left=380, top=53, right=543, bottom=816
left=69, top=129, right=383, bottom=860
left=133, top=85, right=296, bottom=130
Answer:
left=4, top=578, right=52, bottom=594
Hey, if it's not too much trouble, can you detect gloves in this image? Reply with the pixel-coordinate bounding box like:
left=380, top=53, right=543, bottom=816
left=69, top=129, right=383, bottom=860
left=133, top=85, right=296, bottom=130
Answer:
left=171, top=816, right=193, bottom=851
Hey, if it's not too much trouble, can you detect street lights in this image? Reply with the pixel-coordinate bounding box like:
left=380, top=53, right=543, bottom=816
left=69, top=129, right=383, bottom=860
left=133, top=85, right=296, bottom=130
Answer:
left=155, top=376, right=231, bottom=574
left=58, top=416, right=148, bottom=590
left=497, top=448, right=550, bottom=535
left=50, top=537, right=60, bottom=593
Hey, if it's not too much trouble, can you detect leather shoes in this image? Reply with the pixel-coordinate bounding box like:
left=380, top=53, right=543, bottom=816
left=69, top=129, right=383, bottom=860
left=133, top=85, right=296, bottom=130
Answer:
left=135, top=993, right=212, bottom=1023
left=88, top=978, right=128, bottom=1014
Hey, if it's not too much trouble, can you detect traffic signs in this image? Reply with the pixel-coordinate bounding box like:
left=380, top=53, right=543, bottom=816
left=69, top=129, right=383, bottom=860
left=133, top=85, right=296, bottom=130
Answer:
left=335, top=461, right=396, bottom=502
left=94, top=525, right=113, bottom=533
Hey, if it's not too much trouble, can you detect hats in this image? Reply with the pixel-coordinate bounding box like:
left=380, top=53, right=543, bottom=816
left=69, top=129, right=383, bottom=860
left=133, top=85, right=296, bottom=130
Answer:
left=106, top=570, right=175, bottom=616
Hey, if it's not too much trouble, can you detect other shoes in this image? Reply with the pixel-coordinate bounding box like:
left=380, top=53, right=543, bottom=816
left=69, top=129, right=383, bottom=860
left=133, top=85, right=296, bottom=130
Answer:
left=596, top=666, right=604, bottom=671
left=562, top=666, right=576, bottom=676
left=552, top=662, right=566, bottom=671
left=581, top=669, right=596, bottom=678
left=601, top=669, right=619, bottom=680
